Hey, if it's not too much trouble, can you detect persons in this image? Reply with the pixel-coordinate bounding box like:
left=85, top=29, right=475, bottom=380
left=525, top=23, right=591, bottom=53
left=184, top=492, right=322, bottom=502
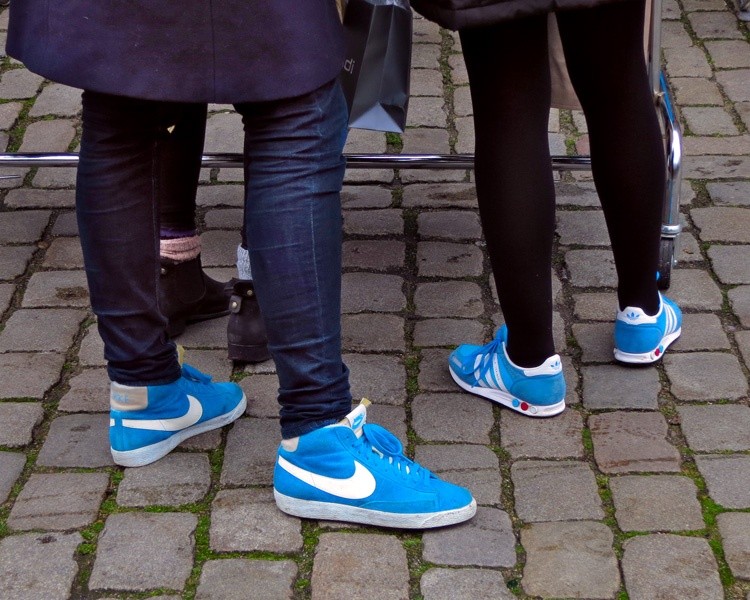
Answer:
left=154, top=0, right=351, bottom=361
left=410, top=0, right=684, bottom=419
left=5, top=0, right=476, bottom=531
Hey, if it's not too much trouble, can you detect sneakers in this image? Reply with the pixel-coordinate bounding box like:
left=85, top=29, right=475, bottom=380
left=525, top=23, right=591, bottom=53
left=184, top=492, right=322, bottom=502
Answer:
left=449, top=324, right=567, bottom=418
left=272, top=401, right=478, bottom=529
left=108, top=365, right=249, bottom=468
left=611, top=287, right=683, bottom=364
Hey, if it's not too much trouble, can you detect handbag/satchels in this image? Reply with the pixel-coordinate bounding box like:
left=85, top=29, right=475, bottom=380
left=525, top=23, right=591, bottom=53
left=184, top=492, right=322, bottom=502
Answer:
left=336, top=0, right=413, bottom=136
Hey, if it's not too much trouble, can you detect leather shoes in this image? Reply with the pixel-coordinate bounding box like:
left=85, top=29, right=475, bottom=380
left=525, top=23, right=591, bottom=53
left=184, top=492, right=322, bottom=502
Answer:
left=149, top=252, right=234, bottom=340
left=225, top=276, right=270, bottom=362
